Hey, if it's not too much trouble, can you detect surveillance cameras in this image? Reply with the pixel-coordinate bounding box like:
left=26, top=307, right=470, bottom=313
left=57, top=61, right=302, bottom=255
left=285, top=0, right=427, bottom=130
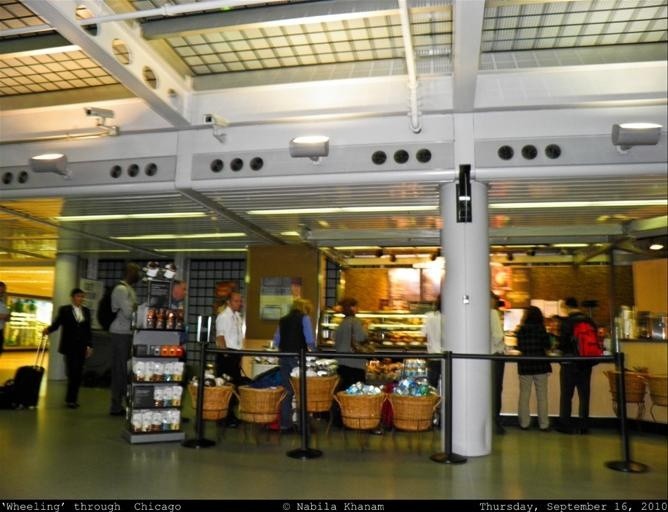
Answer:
left=83, top=106, right=119, bottom=135
left=202, top=112, right=228, bottom=143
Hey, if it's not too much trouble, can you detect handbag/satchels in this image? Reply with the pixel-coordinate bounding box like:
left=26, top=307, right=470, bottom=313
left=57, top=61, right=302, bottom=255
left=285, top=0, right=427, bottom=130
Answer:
left=350, top=317, right=377, bottom=354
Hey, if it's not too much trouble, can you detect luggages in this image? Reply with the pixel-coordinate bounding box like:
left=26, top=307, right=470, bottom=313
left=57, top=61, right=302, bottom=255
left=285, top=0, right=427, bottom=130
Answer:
left=15, top=331, right=50, bottom=409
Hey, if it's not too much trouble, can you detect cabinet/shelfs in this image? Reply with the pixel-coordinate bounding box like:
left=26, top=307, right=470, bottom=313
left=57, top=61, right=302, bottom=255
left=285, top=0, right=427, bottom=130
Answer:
left=318, top=308, right=427, bottom=354
left=617, top=337, right=668, bottom=427
left=125, top=304, right=187, bottom=443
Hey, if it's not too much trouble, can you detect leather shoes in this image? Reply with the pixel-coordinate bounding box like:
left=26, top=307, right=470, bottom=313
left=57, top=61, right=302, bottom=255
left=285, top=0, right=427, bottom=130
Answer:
left=217, top=420, right=239, bottom=428
left=577, top=431, right=592, bottom=435
left=541, top=427, right=551, bottom=432
left=521, top=426, right=530, bottom=430
left=110, top=409, right=126, bottom=417
left=76, top=402, right=80, bottom=407
left=66, top=403, right=77, bottom=409
left=556, top=427, right=572, bottom=434
left=370, top=426, right=383, bottom=436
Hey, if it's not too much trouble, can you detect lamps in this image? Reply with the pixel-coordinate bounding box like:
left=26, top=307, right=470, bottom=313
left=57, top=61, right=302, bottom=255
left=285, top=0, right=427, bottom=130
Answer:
left=293, top=137, right=332, bottom=159
left=31, top=154, right=70, bottom=176
left=612, top=123, right=661, bottom=151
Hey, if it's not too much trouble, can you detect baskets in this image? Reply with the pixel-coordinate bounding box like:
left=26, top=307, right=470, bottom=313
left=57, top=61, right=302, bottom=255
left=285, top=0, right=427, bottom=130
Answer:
left=187, top=382, right=233, bottom=421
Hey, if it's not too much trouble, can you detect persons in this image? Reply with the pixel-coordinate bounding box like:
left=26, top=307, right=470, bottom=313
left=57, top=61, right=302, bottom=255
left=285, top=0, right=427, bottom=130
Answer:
left=271, top=298, right=322, bottom=437
left=547, top=315, right=562, bottom=354
left=420, top=294, right=442, bottom=389
left=554, top=296, right=597, bottom=433
left=515, top=306, right=553, bottom=432
left=42, top=289, right=94, bottom=410
left=330, top=298, right=370, bottom=431
left=169, top=278, right=187, bottom=309
left=490, top=291, right=507, bottom=433
left=213, top=292, right=244, bottom=426
left=106, top=263, right=144, bottom=417
left=0, top=281, right=12, bottom=359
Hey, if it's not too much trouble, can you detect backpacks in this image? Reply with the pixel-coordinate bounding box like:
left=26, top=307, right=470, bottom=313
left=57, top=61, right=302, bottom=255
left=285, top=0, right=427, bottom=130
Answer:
left=566, top=317, right=604, bottom=368
left=96, top=281, right=128, bottom=333
